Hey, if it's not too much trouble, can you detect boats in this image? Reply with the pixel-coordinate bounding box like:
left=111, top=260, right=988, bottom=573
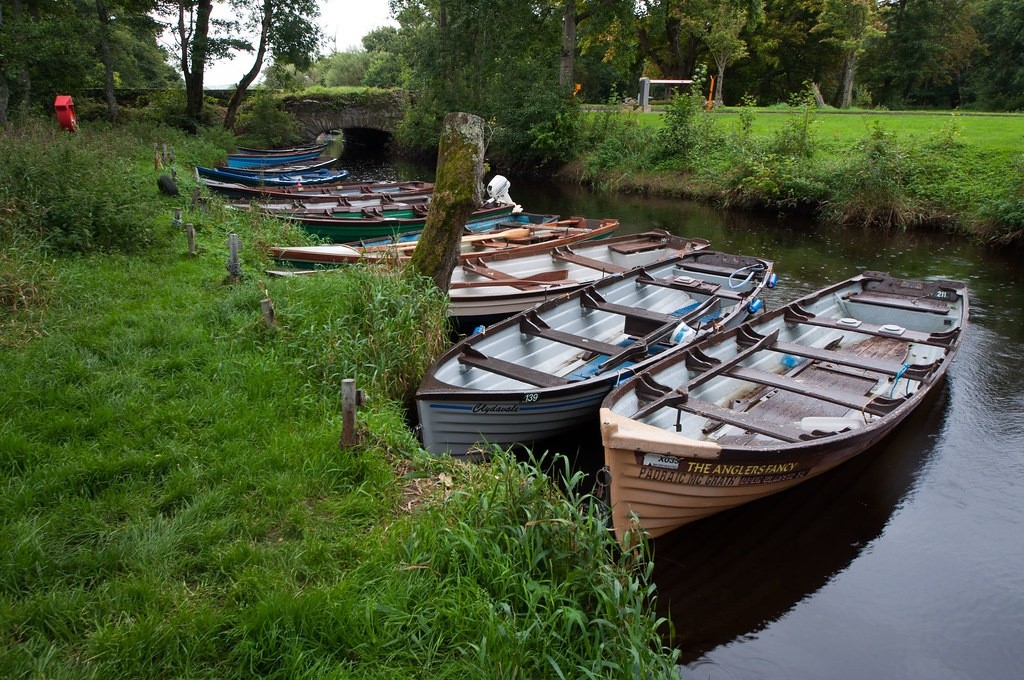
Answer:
left=413, top=249, right=785, bottom=466
left=443, top=226, right=713, bottom=338
left=639, top=372, right=955, bottom=663
left=192, top=138, right=619, bottom=278
left=600, top=266, right=992, bottom=555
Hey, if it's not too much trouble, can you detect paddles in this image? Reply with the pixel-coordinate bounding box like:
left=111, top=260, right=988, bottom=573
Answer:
left=451, top=268, right=579, bottom=288
left=387, top=228, right=530, bottom=247
